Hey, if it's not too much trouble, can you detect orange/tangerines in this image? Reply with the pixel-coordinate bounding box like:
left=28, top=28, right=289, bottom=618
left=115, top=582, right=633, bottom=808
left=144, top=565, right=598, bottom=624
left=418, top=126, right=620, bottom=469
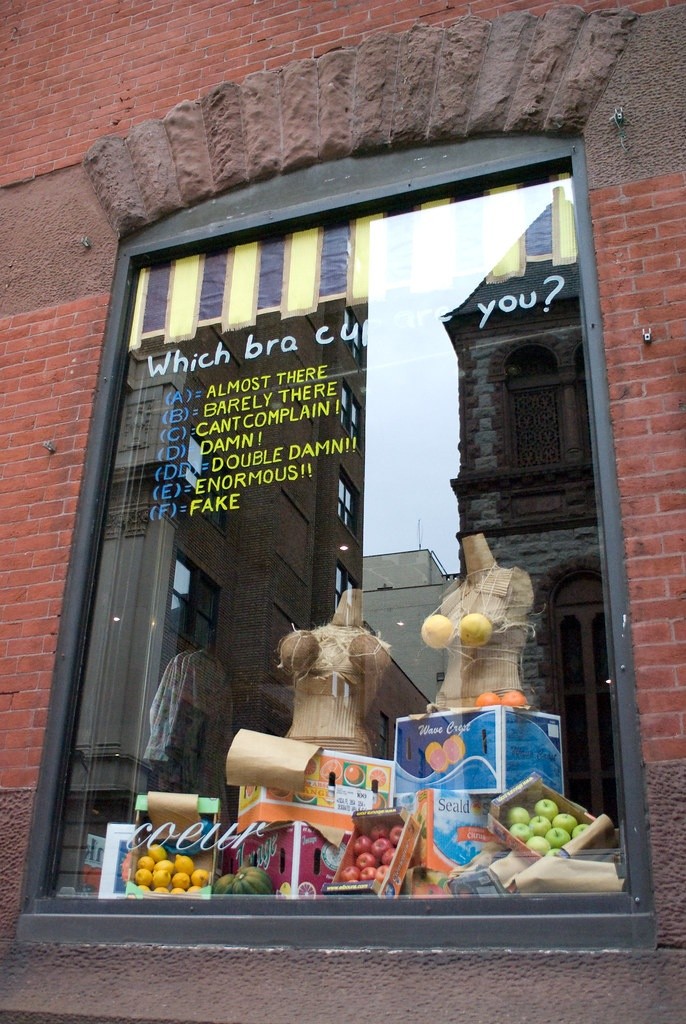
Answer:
left=476, top=691, right=527, bottom=707
left=421, top=613, right=492, bottom=649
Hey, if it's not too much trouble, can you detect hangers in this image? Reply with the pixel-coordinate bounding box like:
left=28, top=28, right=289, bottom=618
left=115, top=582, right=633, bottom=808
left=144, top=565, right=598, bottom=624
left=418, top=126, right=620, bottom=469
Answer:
left=184, top=635, right=221, bottom=668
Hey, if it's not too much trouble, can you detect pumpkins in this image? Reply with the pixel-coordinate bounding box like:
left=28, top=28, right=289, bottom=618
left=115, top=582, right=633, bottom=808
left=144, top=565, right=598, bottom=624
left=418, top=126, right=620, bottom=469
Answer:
left=212, top=866, right=274, bottom=895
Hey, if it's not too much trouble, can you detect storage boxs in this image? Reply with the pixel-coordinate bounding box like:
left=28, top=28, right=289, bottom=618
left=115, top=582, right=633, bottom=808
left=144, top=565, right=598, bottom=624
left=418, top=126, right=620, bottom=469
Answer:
left=125, top=791, right=223, bottom=901
left=223, top=822, right=353, bottom=899
left=237, top=749, right=395, bottom=836
left=487, top=772, right=614, bottom=864
left=98, top=822, right=135, bottom=898
left=394, top=706, right=564, bottom=799
left=320, top=807, right=423, bottom=899
left=401, top=788, right=517, bottom=896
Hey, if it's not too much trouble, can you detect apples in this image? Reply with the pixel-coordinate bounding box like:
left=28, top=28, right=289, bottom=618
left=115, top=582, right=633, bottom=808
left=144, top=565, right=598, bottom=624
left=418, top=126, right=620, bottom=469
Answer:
left=505, top=798, right=591, bottom=859
left=341, top=824, right=404, bottom=884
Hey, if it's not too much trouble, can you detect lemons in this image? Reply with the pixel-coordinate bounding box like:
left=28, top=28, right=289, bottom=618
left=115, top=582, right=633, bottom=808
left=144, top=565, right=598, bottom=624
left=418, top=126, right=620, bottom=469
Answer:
left=134, top=844, right=209, bottom=894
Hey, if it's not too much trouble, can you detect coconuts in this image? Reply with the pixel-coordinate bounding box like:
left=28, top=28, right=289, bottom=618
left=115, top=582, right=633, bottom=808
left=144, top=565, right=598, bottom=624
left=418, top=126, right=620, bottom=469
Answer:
left=347, top=634, right=390, bottom=679
left=279, top=630, right=320, bottom=672
